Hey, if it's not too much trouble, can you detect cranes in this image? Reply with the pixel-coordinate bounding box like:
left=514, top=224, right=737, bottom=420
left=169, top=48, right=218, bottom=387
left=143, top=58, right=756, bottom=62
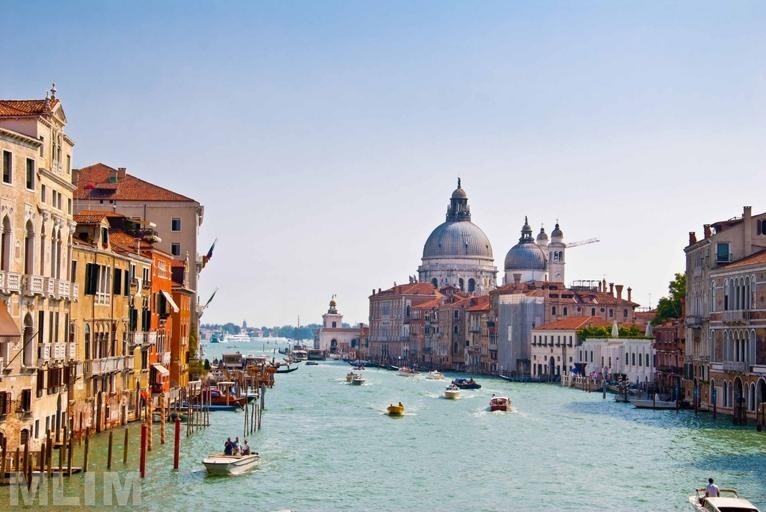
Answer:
left=566, top=237, right=601, bottom=249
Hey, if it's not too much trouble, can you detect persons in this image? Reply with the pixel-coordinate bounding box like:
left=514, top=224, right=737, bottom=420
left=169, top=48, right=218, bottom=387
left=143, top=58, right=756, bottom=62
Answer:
left=451, top=377, right=474, bottom=389
left=704, top=478, right=720, bottom=497
left=398, top=401, right=403, bottom=407
left=591, top=371, right=604, bottom=385
left=490, top=394, right=498, bottom=405
left=224, top=435, right=250, bottom=456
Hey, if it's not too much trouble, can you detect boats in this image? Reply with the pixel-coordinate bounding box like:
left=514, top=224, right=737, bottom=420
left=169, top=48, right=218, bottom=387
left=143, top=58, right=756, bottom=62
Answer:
left=212, top=353, right=298, bottom=387
left=445, top=379, right=481, bottom=399
left=202, top=452, right=261, bottom=476
left=348, top=359, right=371, bottom=366
left=346, top=375, right=364, bottom=385
left=291, top=349, right=323, bottom=360
left=615, top=394, right=680, bottom=409
left=387, top=404, right=403, bottom=414
left=689, top=488, right=759, bottom=512
left=500, top=374, right=560, bottom=382
left=399, top=368, right=419, bottom=376
left=490, top=397, right=508, bottom=411
left=193, top=382, right=257, bottom=405
left=427, top=371, right=443, bottom=379
